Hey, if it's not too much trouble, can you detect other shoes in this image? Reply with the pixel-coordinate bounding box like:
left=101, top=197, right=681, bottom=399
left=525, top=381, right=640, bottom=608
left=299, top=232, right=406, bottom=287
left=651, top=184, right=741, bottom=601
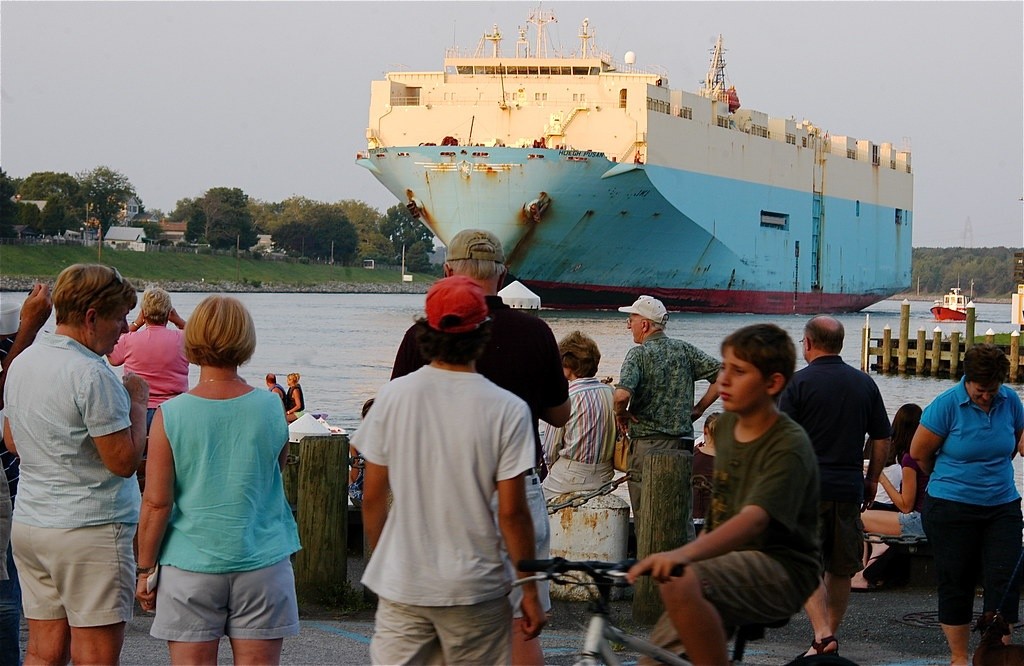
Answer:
left=850, top=587, right=870, bottom=592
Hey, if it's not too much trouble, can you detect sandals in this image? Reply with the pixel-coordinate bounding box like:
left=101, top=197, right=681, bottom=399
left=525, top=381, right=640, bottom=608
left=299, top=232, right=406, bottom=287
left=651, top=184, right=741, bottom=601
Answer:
left=795, top=636, right=839, bottom=659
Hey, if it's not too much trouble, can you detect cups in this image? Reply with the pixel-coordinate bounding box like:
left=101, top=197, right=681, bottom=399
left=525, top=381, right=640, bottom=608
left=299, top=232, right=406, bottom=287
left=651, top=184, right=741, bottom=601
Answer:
left=0, top=302, right=22, bottom=335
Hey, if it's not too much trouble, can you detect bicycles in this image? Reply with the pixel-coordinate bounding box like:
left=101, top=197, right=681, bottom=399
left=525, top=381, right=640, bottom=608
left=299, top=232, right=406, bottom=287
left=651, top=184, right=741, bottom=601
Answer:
left=510, top=556, right=859, bottom=666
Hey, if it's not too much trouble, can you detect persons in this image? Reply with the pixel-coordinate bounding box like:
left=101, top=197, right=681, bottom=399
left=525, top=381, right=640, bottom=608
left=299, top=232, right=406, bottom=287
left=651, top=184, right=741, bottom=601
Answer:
left=910, top=341, right=1024, bottom=666
left=0, top=281, right=54, bottom=666
left=849, top=404, right=936, bottom=594
left=776, top=314, right=892, bottom=663
left=3, top=262, right=149, bottom=666
left=690, top=411, right=721, bottom=517
left=625, top=322, right=822, bottom=666
left=612, top=295, right=723, bottom=544
left=107, top=288, right=190, bottom=453
left=136, top=295, right=305, bottom=666
left=265, top=228, right=572, bottom=666
left=541, top=330, right=616, bottom=503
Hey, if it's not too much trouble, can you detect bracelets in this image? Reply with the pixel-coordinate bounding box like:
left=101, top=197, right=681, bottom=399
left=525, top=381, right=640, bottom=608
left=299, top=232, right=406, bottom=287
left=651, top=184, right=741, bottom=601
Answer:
left=287, top=410, right=291, bottom=414
left=136, top=565, right=156, bottom=574
left=612, top=409, right=624, bottom=416
left=132, top=321, right=139, bottom=328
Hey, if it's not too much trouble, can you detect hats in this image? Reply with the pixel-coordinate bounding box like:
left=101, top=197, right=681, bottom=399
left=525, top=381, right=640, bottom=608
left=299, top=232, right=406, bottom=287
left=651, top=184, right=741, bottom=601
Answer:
left=426, top=275, right=491, bottom=332
left=444, top=229, right=505, bottom=277
left=618, top=295, right=669, bottom=324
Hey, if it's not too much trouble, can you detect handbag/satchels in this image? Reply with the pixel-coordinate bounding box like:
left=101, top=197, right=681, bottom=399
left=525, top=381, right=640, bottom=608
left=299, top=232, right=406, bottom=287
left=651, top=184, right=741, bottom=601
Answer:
left=615, top=430, right=631, bottom=471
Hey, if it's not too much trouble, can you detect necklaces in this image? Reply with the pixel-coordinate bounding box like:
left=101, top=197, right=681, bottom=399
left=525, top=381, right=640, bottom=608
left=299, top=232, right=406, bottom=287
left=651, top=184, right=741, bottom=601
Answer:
left=199, top=378, right=240, bottom=383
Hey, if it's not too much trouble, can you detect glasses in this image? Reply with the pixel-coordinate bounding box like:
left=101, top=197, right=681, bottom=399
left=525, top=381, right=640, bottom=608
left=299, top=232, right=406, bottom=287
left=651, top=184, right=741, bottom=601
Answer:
left=799, top=338, right=805, bottom=344
left=626, top=317, right=644, bottom=325
left=84, top=266, right=123, bottom=304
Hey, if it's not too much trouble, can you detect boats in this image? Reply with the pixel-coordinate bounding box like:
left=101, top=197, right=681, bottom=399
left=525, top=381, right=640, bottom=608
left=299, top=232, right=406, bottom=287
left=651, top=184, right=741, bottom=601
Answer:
left=929, top=287, right=978, bottom=321
left=355, top=1, right=913, bottom=315
